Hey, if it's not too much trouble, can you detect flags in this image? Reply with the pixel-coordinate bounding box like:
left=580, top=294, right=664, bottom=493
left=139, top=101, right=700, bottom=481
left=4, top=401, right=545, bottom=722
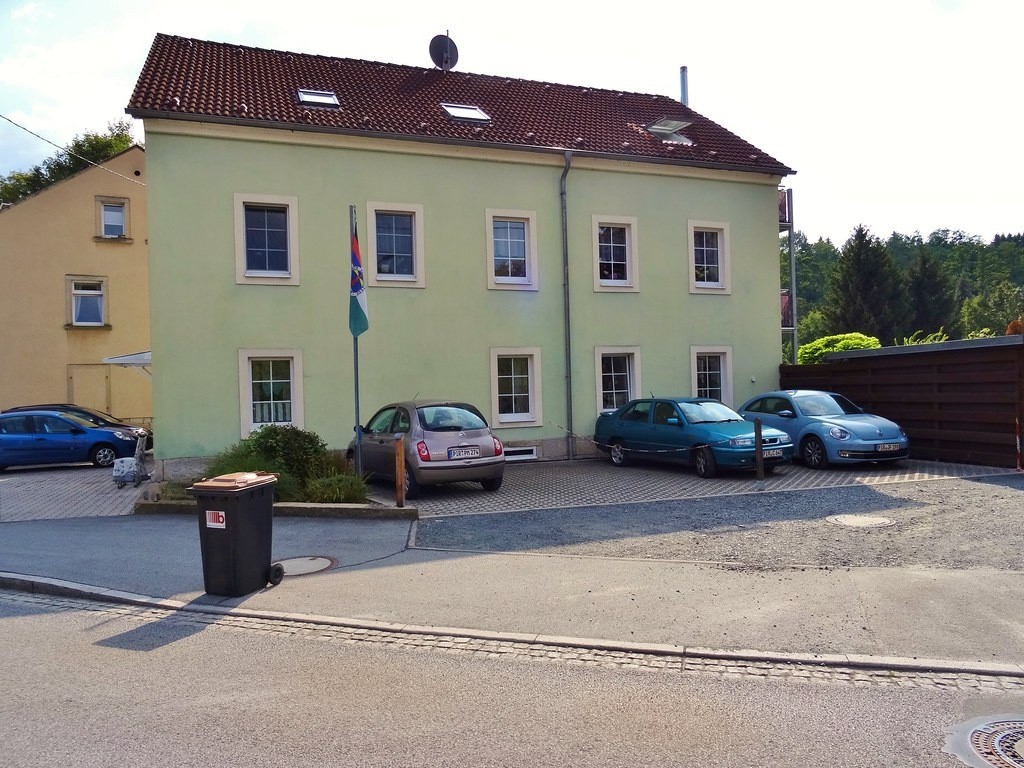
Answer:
left=348, top=219, right=369, bottom=338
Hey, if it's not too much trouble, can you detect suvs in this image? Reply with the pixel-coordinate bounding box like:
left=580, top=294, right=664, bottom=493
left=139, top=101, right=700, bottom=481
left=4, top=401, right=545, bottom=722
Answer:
left=1, top=402, right=154, bottom=452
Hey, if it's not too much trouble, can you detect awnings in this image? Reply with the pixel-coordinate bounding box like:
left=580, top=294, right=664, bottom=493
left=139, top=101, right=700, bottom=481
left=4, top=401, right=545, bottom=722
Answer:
left=103, top=350, right=151, bottom=379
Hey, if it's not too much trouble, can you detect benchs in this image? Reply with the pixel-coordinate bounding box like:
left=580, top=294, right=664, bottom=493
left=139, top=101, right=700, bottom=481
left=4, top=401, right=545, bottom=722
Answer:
left=113, top=435, right=149, bottom=482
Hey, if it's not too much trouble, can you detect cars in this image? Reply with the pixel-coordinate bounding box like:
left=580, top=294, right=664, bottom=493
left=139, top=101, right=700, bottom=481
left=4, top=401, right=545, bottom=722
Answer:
left=0, top=411, right=138, bottom=472
left=346, top=399, right=505, bottom=500
left=593, top=397, right=795, bottom=480
left=737, top=390, right=911, bottom=471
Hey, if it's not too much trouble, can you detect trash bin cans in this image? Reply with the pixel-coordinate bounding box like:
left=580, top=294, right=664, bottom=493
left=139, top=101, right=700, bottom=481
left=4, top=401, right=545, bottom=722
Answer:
left=186, top=472, right=285, bottom=598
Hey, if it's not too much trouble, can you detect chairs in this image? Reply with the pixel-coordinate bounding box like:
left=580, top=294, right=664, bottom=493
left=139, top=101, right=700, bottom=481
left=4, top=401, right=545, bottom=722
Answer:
left=431, top=408, right=452, bottom=427
